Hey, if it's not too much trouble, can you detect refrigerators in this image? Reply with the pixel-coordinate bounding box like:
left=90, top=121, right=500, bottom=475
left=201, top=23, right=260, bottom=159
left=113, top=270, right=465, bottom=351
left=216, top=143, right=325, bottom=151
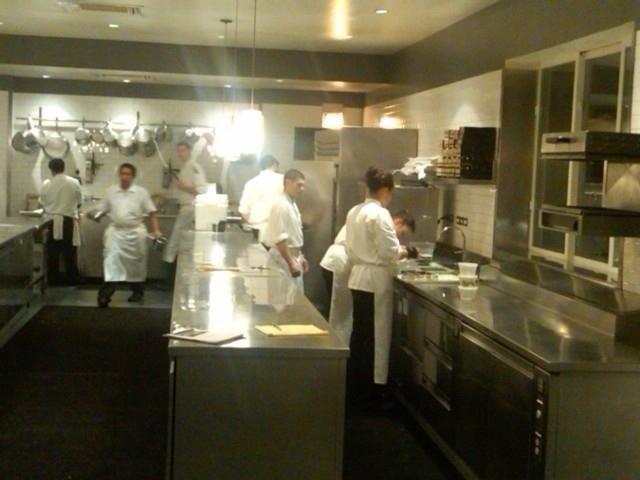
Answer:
left=312, top=126, right=419, bottom=252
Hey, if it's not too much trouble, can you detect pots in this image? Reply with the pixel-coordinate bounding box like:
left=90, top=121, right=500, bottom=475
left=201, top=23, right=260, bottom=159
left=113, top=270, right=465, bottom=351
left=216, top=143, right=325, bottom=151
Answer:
left=9, top=106, right=174, bottom=159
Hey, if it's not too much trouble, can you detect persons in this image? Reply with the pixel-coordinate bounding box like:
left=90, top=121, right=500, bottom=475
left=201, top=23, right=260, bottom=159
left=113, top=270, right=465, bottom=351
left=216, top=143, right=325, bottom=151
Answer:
left=265, top=170, right=310, bottom=295
left=343, top=166, right=419, bottom=411
left=151, top=142, right=206, bottom=290
left=37, top=159, right=84, bottom=290
left=237, top=155, right=285, bottom=252
left=87, top=164, right=161, bottom=308
left=320, top=210, right=415, bottom=348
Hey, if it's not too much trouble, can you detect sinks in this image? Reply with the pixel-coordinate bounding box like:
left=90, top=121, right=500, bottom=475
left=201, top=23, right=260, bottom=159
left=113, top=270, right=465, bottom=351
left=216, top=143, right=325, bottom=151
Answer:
left=417, top=261, right=430, bottom=267
left=418, top=267, right=447, bottom=273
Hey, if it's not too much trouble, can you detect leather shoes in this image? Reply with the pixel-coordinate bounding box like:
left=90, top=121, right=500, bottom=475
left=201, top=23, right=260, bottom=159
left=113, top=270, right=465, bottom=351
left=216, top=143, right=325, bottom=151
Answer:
left=128, top=294, right=143, bottom=302
left=98, top=297, right=109, bottom=306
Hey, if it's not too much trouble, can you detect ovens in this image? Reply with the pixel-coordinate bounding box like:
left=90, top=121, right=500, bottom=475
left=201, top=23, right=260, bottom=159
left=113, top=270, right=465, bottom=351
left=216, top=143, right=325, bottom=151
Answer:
left=419, top=313, right=548, bottom=478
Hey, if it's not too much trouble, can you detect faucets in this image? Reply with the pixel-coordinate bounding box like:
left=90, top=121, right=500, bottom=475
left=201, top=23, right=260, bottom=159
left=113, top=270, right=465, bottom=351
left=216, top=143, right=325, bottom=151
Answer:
left=438, top=224, right=466, bottom=254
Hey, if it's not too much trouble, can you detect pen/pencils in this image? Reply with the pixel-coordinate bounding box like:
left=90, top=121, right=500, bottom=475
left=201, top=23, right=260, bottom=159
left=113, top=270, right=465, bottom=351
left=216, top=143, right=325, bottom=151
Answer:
left=271, top=322, right=283, bottom=331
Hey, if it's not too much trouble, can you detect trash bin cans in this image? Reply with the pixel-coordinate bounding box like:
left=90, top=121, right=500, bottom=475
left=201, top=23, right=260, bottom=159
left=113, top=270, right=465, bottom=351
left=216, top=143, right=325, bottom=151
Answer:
left=46, top=239, right=77, bottom=286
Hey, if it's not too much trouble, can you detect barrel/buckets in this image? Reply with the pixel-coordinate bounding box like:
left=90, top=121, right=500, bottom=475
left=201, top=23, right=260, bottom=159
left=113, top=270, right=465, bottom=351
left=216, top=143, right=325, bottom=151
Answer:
left=191, top=193, right=229, bottom=231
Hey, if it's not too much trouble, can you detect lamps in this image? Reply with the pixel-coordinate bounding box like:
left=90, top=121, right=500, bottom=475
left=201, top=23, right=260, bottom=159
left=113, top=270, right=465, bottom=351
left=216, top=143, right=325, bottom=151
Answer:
left=321, top=99, right=347, bottom=132
left=205, top=0, right=265, bottom=161
